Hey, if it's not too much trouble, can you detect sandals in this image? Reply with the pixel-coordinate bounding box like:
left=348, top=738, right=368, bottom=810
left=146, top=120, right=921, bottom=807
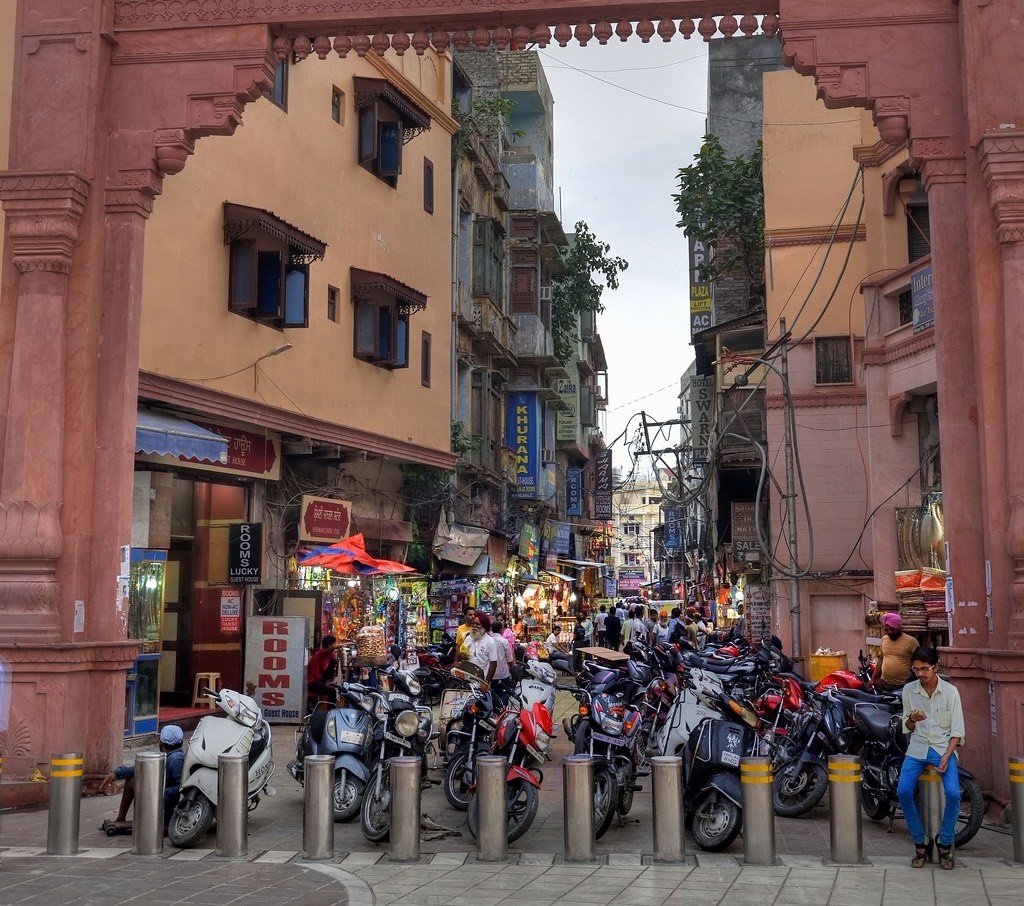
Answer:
left=911, top=835, right=934, bottom=868
left=935, top=833, right=954, bottom=869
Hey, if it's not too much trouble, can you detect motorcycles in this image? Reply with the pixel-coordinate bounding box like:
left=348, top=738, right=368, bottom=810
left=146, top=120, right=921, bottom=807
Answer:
left=289, top=615, right=986, bottom=853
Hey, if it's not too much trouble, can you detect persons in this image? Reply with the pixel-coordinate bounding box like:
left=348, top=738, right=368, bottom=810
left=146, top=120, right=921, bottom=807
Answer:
left=308, top=636, right=345, bottom=711
left=896, top=644, right=966, bottom=870
left=99, top=724, right=185, bottom=831
left=454, top=597, right=746, bottom=711
left=865, top=612, right=921, bottom=696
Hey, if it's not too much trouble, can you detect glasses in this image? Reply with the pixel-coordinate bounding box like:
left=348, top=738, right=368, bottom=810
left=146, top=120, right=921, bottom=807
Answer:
left=911, top=664, right=935, bottom=672
left=882, top=626, right=895, bottom=631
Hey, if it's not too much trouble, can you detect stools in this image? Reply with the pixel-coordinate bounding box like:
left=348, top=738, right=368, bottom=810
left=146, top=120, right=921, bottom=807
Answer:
left=191, top=673, right=224, bottom=711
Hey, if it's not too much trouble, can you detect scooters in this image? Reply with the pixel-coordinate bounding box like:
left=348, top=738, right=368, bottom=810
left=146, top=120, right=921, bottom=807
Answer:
left=168, top=686, right=276, bottom=850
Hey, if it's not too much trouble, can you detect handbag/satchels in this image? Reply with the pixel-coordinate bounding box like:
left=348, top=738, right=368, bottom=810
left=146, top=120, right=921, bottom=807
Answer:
left=667, top=619, right=689, bottom=643
left=623, top=641, right=633, bottom=655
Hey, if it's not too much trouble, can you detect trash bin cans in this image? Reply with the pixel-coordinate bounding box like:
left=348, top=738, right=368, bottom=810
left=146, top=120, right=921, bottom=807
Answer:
left=806, top=651, right=849, bottom=683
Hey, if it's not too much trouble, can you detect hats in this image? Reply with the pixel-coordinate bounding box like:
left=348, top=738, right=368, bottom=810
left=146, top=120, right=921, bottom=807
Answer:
left=633, top=605, right=642, bottom=613
left=160, top=725, right=184, bottom=745
left=628, top=597, right=634, bottom=602
left=881, top=613, right=902, bottom=629
left=575, top=616, right=583, bottom=623
left=671, top=608, right=683, bottom=614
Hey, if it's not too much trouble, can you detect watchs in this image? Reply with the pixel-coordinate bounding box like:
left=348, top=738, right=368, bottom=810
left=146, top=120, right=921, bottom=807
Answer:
left=908, top=714, right=916, bottom=724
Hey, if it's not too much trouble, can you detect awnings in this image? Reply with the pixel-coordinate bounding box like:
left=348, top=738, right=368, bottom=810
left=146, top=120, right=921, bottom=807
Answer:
left=134, top=402, right=230, bottom=468
left=543, top=569, right=578, bottom=583
left=558, top=559, right=611, bottom=578
